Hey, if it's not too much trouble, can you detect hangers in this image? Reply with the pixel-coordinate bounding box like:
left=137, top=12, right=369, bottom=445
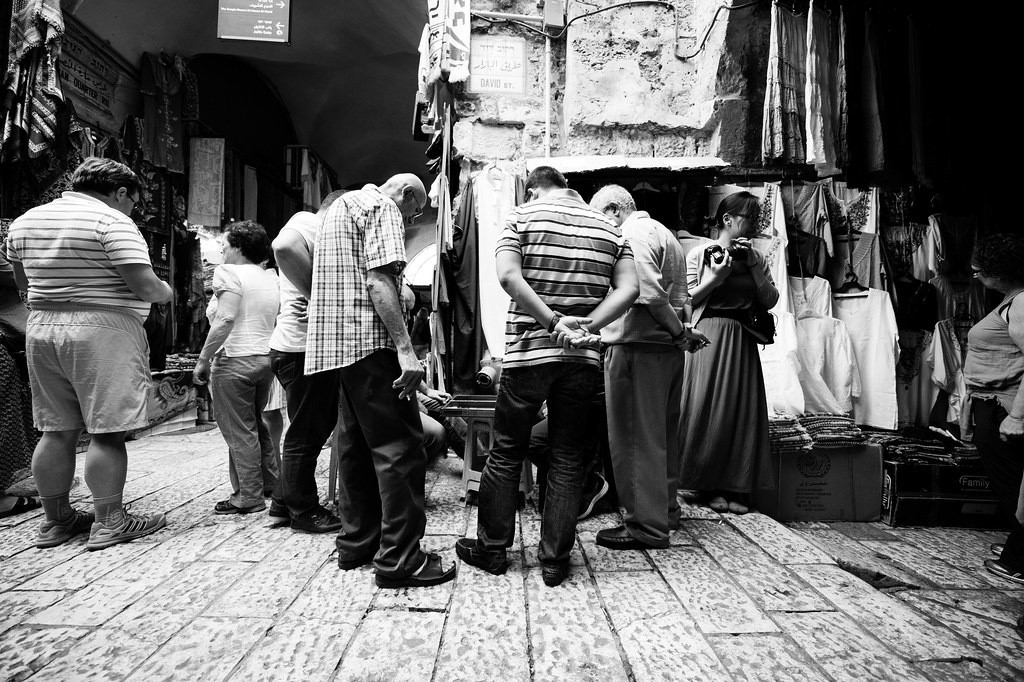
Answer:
left=797, top=298, right=821, bottom=319
left=944, top=260, right=971, bottom=288
left=832, top=271, right=869, bottom=298
left=952, top=302, right=978, bottom=328
left=881, top=203, right=911, bottom=229
left=786, top=214, right=806, bottom=238
left=831, top=216, right=863, bottom=244
left=774, top=0, right=840, bottom=23
left=894, top=262, right=920, bottom=284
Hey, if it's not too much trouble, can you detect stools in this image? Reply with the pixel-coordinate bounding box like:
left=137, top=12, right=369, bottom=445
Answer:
left=459, top=415, right=537, bottom=511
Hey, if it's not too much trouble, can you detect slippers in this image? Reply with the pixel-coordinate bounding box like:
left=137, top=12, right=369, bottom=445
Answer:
left=0, top=494, right=42, bottom=519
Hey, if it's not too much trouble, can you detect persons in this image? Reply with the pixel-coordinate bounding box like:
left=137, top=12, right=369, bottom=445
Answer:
left=191, top=172, right=456, bottom=588
left=455, top=165, right=777, bottom=588
left=962, top=234, right=1023, bottom=583
left=6, top=158, right=174, bottom=550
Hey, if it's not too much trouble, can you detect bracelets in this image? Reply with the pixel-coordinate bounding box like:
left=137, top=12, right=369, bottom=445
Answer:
left=548, top=316, right=560, bottom=333
left=674, top=323, right=685, bottom=338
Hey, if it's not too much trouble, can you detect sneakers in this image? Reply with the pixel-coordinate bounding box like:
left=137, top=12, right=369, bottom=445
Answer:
left=36, top=507, right=95, bottom=548
left=87, top=503, right=166, bottom=549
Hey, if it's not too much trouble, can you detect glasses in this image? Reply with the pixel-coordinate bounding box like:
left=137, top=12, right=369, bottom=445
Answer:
left=128, top=194, right=138, bottom=210
left=733, top=213, right=759, bottom=223
left=971, top=269, right=983, bottom=276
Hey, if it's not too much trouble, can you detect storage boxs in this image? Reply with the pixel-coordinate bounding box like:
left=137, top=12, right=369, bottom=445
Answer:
left=755, top=444, right=1006, bottom=530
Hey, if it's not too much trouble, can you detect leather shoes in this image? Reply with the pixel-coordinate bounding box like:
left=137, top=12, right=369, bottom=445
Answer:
left=596, top=525, right=669, bottom=549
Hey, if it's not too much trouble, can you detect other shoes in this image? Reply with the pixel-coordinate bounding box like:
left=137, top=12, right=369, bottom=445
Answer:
left=730, top=499, right=748, bottom=513
left=991, top=543, right=1004, bottom=555
left=291, top=507, right=342, bottom=533
left=215, top=500, right=266, bottom=513
left=269, top=501, right=289, bottom=517
left=985, top=560, right=1024, bottom=584
left=543, top=569, right=563, bottom=586
left=456, top=538, right=507, bottom=575
left=710, top=495, right=728, bottom=509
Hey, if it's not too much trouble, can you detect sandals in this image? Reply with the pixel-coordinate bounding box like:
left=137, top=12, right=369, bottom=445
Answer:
left=338, top=556, right=372, bottom=570
left=376, top=552, right=456, bottom=589
